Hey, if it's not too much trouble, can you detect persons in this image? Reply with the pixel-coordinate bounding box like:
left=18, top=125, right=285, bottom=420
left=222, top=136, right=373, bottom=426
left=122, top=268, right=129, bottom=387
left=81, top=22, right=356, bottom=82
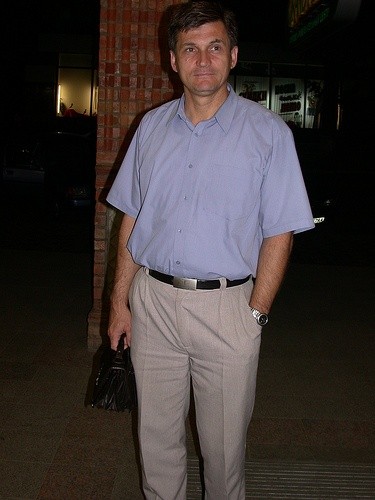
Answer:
left=105, top=0, right=315, bottom=500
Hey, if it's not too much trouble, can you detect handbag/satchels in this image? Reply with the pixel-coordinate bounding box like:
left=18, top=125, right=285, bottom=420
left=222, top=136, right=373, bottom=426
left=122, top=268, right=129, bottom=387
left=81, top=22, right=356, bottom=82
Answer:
left=90, top=332, right=136, bottom=415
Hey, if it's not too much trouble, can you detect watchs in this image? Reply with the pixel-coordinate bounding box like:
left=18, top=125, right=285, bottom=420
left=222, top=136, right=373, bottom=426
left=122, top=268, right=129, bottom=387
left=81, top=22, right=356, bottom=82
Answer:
left=248, top=306, right=269, bottom=326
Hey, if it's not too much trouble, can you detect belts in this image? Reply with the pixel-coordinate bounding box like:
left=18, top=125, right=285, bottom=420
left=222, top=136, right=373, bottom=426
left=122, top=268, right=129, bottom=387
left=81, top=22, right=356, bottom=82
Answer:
left=149, top=268, right=252, bottom=290
